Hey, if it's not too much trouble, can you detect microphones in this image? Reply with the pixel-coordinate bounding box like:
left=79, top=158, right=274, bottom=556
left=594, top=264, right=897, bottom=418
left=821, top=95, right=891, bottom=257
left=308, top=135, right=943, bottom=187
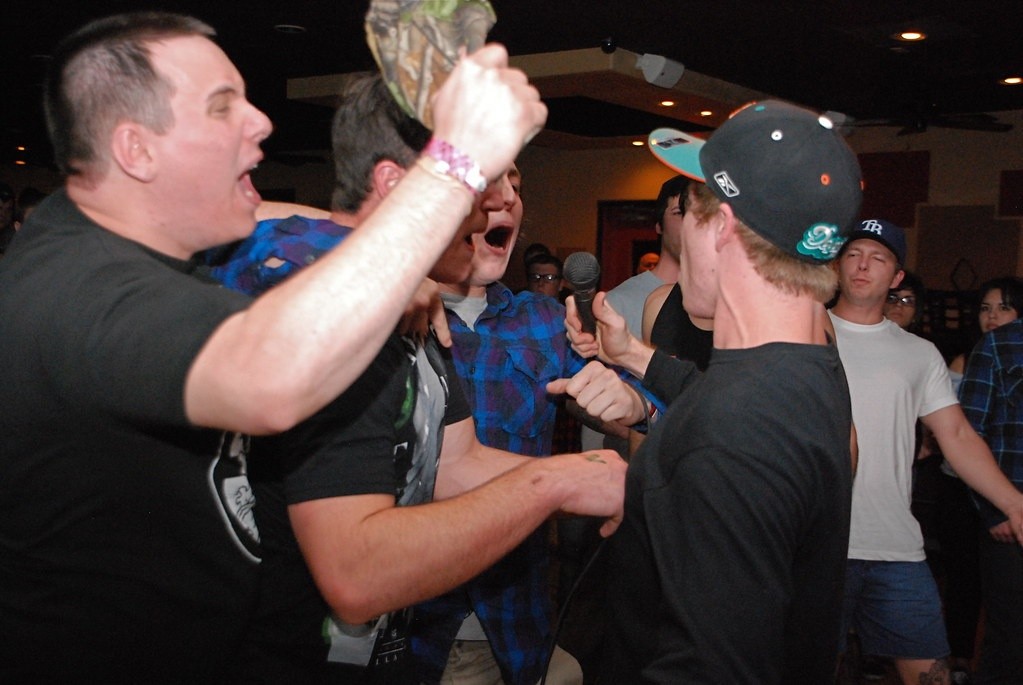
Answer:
left=561, top=252, right=599, bottom=360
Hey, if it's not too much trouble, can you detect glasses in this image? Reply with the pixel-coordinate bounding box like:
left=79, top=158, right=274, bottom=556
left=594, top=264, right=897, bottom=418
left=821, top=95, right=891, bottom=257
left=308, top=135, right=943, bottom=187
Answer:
left=524, top=272, right=560, bottom=282
left=886, top=295, right=918, bottom=308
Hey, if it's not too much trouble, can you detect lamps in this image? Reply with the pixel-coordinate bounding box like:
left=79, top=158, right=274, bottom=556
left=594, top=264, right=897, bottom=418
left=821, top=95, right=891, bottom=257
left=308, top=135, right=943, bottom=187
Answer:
left=635, top=53, right=685, bottom=89
left=825, top=111, right=856, bottom=137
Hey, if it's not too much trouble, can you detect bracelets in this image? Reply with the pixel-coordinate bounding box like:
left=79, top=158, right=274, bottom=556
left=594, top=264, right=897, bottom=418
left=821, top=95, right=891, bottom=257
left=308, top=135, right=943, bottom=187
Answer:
left=419, top=136, right=488, bottom=212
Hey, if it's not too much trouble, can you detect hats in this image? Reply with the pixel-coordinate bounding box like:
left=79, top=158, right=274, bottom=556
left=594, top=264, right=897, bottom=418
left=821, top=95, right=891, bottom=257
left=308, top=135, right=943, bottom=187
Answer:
left=365, top=0, right=496, bottom=132
left=835, top=220, right=906, bottom=271
left=647, top=101, right=863, bottom=262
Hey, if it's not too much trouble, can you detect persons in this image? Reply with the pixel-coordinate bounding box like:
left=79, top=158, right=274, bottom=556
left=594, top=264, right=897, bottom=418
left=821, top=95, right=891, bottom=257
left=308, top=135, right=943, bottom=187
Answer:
left=193, top=75, right=627, bottom=685
left=202, top=158, right=663, bottom=685
left=528, top=101, right=863, bottom=685
left=504, top=174, right=714, bottom=587
left=825, top=215, right=1023, bottom=685
left=0, top=11, right=547, bottom=685
